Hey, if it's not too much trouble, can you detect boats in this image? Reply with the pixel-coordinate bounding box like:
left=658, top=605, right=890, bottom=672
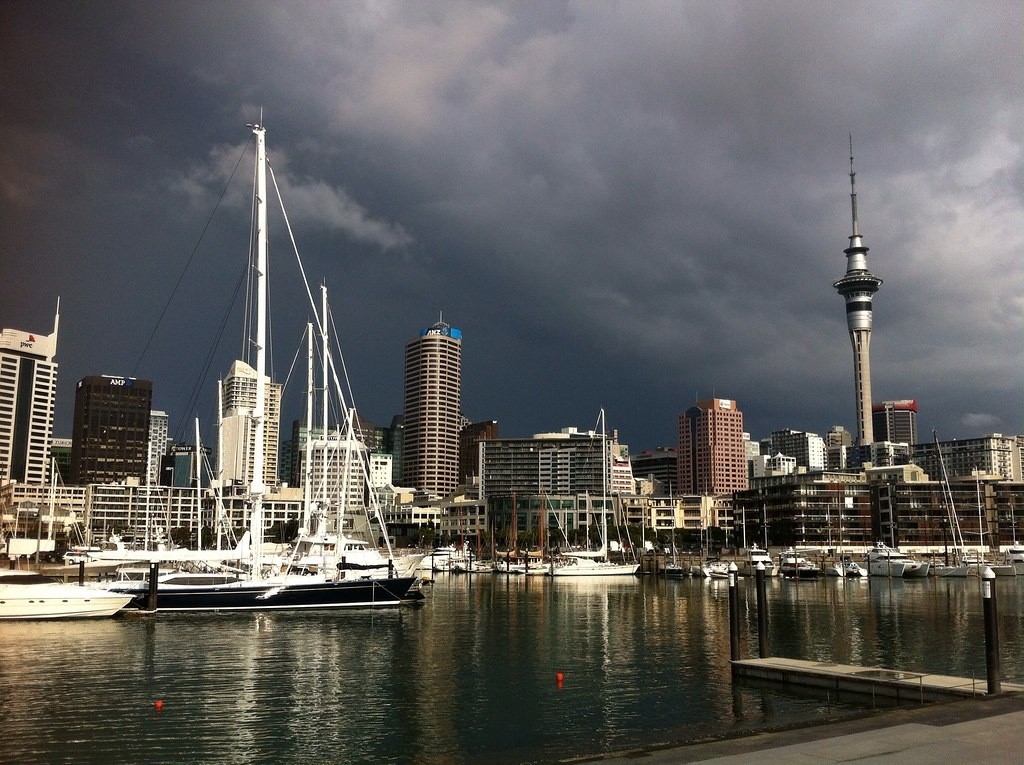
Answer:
left=1, top=100, right=1024, bottom=624
